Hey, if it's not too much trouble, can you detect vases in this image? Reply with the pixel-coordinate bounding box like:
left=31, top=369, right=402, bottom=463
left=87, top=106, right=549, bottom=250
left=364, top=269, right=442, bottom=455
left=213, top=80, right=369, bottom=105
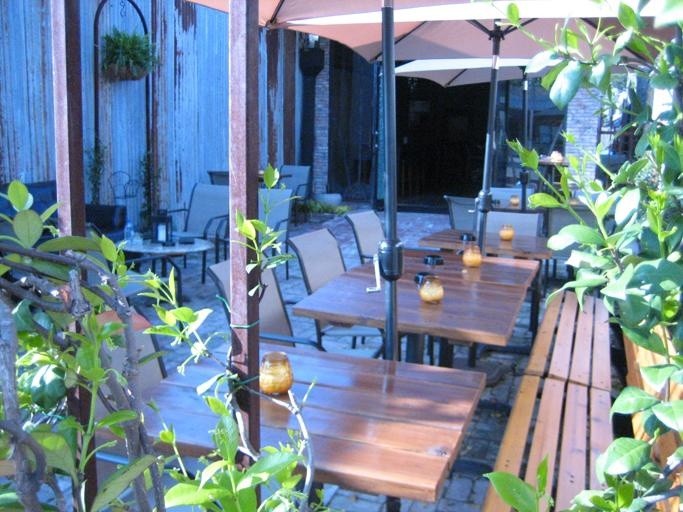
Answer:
left=308, top=192, right=342, bottom=207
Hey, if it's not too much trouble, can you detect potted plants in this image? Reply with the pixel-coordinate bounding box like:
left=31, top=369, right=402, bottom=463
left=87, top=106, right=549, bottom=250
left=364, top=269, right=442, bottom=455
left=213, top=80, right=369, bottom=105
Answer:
left=95, top=25, right=162, bottom=81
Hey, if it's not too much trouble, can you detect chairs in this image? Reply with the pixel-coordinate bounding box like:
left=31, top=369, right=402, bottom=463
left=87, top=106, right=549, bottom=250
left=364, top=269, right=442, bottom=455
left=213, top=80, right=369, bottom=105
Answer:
left=443, top=187, right=617, bottom=297
left=166, top=163, right=311, bottom=286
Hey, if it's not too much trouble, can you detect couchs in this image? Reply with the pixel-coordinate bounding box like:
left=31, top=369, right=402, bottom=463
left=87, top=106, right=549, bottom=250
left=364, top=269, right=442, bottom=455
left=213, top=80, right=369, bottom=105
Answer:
left=0, top=178, right=125, bottom=305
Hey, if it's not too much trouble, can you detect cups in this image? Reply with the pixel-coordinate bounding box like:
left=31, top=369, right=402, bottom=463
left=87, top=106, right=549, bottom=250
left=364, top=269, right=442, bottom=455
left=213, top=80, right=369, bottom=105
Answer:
left=499, top=224, right=515, bottom=241
left=510, top=196, right=520, bottom=206
left=259, top=351, right=294, bottom=394
left=419, top=276, right=444, bottom=305
left=462, top=245, right=482, bottom=267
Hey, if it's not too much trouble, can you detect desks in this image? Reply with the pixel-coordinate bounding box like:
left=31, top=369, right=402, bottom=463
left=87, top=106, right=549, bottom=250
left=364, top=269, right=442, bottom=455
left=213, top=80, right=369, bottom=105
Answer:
left=92, top=227, right=554, bottom=502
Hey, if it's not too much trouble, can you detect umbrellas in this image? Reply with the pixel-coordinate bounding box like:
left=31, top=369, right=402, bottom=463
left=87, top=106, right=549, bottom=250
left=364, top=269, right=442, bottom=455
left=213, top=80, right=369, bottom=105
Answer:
left=381, top=59, right=640, bottom=213
left=285, top=17, right=676, bottom=361
left=189, top=0, right=676, bottom=361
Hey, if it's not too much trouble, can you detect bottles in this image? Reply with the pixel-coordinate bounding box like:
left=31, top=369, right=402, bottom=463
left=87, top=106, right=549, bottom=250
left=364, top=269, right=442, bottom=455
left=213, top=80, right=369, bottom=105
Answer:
left=124, top=220, right=134, bottom=247
